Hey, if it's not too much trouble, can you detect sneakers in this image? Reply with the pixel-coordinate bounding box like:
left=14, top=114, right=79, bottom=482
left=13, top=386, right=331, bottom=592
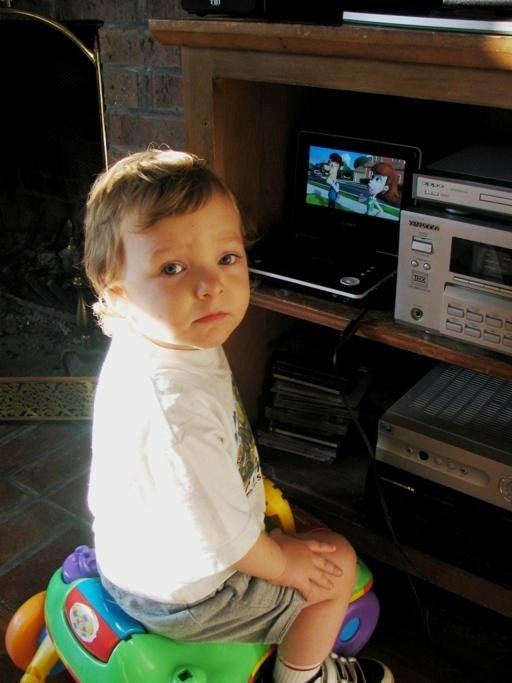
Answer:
left=259, top=652, right=396, bottom=683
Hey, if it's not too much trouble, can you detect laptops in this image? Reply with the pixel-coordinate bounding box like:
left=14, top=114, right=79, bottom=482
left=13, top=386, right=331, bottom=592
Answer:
left=244, top=130, right=423, bottom=308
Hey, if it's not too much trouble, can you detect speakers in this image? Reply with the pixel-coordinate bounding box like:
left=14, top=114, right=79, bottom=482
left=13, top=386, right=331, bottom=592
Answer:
left=360, top=458, right=512, bottom=592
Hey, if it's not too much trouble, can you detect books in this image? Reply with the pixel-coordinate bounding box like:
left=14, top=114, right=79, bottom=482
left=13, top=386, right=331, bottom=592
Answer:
left=255, top=353, right=354, bottom=464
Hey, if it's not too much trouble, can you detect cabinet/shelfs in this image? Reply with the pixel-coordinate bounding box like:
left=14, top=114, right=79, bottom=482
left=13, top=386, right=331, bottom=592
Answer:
left=150, top=18, right=511, bottom=620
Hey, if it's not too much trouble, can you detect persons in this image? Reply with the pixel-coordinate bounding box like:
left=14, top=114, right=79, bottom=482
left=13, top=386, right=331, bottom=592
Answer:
left=79, top=148, right=398, bottom=683
